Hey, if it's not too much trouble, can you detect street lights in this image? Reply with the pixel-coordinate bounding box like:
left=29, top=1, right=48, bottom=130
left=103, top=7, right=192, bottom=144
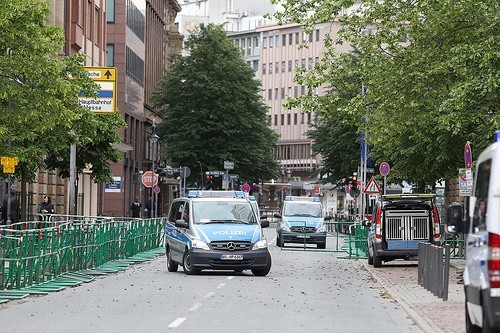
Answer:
left=149, top=123, right=160, bottom=217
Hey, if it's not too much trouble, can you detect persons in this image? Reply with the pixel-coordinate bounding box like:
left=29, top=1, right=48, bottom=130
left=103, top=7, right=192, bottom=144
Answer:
left=130, top=197, right=141, bottom=219
left=475, top=199, right=485, bottom=228
left=40, top=196, right=55, bottom=225
left=147, top=198, right=156, bottom=217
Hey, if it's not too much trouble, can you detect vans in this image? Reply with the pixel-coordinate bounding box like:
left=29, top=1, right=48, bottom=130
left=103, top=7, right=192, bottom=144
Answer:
left=274, top=196, right=332, bottom=249
left=165, top=190, right=271, bottom=276
left=362, top=194, right=441, bottom=268
left=462, top=143, right=500, bottom=333
